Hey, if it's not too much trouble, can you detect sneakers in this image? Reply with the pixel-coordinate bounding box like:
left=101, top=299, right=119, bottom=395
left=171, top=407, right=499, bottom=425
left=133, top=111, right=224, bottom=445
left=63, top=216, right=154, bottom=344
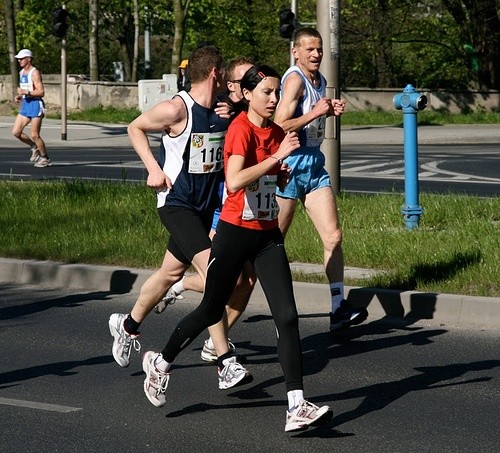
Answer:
left=284, top=399, right=333, bottom=432
left=142, top=350, right=171, bottom=407
left=218, top=356, right=253, bottom=390
left=30, top=149, right=40, bottom=162
left=201, top=339, right=236, bottom=362
left=34, top=157, right=52, bottom=167
left=154, top=287, right=183, bottom=314
left=329, top=299, right=369, bottom=332
left=109, top=313, right=142, bottom=367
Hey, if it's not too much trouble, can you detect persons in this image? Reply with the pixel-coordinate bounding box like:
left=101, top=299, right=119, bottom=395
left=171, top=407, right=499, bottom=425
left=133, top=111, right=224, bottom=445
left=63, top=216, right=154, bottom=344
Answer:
left=108, top=45, right=253, bottom=389
left=143, top=62, right=333, bottom=430
left=154, top=74, right=246, bottom=360
left=275, top=25, right=359, bottom=331
left=177, top=59, right=189, bottom=90
left=12, top=49, right=52, bottom=167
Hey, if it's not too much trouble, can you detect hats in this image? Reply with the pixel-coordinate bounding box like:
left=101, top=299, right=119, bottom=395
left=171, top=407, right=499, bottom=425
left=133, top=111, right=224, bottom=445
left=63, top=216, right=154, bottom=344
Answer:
left=179, top=60, right=188, bottom=69
left=15, top=49, right=32, bottom=59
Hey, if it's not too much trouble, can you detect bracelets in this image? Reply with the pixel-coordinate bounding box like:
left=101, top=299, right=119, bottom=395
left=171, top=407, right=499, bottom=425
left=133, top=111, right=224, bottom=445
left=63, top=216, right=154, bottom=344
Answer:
left=270, top=156, right=282, bottom=164
left=28, top=90, right=30, bottom=96
